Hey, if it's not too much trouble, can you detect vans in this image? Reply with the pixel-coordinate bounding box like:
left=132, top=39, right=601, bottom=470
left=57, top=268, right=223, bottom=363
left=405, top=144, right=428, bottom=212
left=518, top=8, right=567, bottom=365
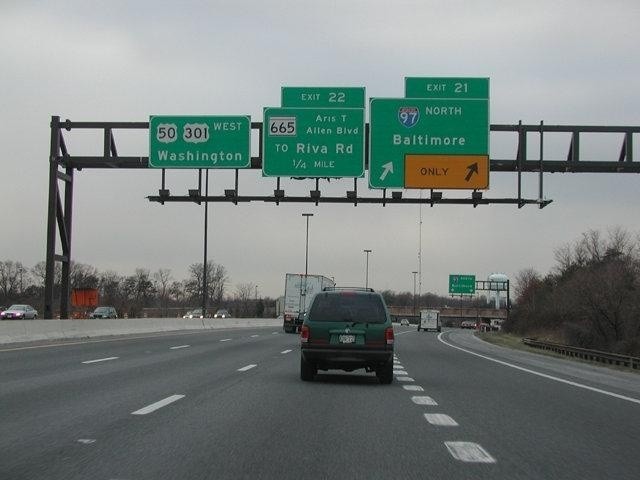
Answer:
left=301, top=286, right=394, bottom=382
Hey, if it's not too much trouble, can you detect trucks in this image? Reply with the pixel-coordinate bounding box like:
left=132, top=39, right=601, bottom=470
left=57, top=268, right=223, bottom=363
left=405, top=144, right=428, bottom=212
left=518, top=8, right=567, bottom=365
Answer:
left=418, top=310, right=442, bottom=331
left=283, top=272, right=335, bottom=335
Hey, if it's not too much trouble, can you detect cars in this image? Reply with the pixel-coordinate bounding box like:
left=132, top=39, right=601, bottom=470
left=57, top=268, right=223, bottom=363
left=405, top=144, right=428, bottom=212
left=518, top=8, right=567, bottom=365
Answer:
left=183, top=309, right=230, bottom=319
left=0, top=305, right=38, bottom=320
left=401, top=319, right=409, bottom=326
left=460, top=320, right=477, bottom=328
left=89, top=307, right=118, bottom=320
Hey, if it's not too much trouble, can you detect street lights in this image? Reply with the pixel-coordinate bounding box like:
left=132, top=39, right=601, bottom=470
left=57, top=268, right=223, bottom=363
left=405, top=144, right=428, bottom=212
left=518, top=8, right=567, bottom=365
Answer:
left=302, top=214, right=313, bottom=274
left=363, top=249, right=372, bottom=292
left=412, top=271, right=418, bottom=321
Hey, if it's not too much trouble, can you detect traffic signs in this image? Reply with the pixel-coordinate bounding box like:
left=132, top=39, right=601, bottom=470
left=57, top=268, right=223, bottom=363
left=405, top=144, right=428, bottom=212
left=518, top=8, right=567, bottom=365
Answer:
left=448, top=274, right=475, bottom=296
